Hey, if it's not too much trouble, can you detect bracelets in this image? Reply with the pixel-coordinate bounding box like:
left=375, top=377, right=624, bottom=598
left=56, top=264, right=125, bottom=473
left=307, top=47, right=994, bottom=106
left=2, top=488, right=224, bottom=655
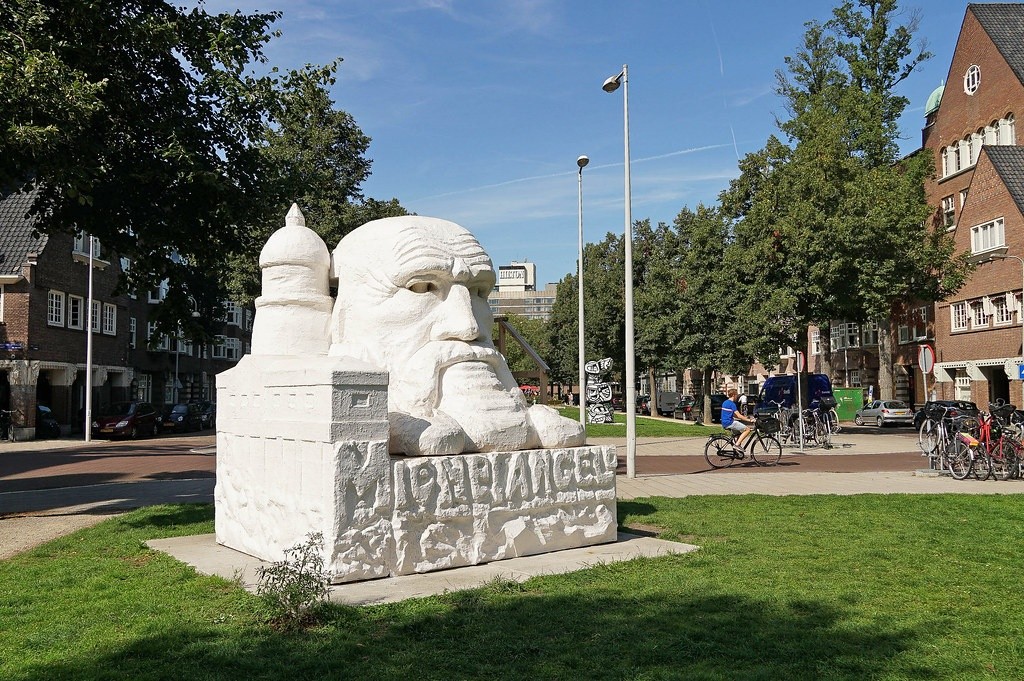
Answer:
left=750, top=419, right=752, bottom=423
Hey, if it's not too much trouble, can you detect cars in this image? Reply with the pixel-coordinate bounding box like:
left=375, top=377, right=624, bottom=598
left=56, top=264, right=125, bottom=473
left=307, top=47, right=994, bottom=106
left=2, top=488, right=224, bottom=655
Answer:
left=160, top=400, right=216, bottom=434
left=855, top=399, right=916, bottom=428
left=36, top=405, right=62, bottom=439
left=672, top=399, right=695, bottom=420
left=609, top=393, right=648, bottom=416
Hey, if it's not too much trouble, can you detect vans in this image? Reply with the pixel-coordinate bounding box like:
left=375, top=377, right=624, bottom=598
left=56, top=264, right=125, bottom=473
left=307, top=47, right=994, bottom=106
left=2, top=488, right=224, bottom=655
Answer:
left=752, top=371, right=833, bottom=422
left=646, top=391, right=680, bottom=416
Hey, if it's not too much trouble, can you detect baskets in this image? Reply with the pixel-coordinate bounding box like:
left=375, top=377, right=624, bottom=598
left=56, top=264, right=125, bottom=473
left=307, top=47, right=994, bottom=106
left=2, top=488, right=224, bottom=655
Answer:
left=761, top=417, right=781, bottom=433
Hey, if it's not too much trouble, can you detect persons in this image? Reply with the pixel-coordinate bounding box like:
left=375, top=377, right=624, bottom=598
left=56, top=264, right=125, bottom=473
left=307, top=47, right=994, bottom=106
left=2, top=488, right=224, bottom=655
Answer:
left=522, top=389, right=575, bottom=406
left=721, top=389, right=756, bottom=459
left=328, top=217, right=537, bottom=458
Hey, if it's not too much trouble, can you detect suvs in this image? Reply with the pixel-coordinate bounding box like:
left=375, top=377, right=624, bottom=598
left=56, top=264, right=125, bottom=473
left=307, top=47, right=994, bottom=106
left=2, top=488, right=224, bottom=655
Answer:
left=91, top=400, right=161, bottom=440
left=915, top=400, right=983, bottom=435
left=688, top=393, right=731, bottom=425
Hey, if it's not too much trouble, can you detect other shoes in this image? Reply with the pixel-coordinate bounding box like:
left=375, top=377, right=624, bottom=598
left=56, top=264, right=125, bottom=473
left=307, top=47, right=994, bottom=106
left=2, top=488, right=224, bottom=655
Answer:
left=732, top=444, right=746, bottom=452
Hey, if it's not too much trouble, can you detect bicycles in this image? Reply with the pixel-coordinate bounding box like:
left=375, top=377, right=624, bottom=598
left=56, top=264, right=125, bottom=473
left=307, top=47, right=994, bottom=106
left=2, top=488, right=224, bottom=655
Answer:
left=0, top=409, right=24, bottom=442
left=704, top=413, right=782, bottom=470
left=919, top=401, right=1024, bottom=482
left=771, top=389, right=841, bottom=452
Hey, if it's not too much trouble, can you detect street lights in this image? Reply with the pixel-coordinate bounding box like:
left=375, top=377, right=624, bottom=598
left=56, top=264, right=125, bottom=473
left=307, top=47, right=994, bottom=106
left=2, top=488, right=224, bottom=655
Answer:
left=601, top=64, right=634, bottom=479
left=575, top=154, right=591, bottom=435
